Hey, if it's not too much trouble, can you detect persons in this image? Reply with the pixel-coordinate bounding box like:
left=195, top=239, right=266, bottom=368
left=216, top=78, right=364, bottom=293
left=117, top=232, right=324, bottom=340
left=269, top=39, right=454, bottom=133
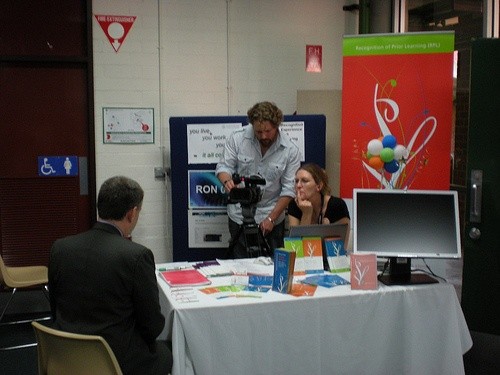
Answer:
left=46, top=175, right=174, bottom=375
left=215, top=101, right=300, bottom=259
left=287, top=164, right=351, bottom=251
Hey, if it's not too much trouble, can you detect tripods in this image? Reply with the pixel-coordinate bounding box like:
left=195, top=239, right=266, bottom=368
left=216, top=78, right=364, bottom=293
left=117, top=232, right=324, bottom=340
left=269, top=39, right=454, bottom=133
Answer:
left=225, top=204, right=272, bottom=260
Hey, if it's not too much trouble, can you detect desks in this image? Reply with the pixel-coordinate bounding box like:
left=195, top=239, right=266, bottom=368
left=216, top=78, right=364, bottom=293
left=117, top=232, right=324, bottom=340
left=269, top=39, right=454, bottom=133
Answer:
left=154, top=258, right=473, bottom=375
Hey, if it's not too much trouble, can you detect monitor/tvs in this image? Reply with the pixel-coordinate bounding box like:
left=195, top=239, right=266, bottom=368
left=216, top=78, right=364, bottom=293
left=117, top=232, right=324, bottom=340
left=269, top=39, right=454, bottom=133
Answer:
left=351, top=188, right=462, bottom=286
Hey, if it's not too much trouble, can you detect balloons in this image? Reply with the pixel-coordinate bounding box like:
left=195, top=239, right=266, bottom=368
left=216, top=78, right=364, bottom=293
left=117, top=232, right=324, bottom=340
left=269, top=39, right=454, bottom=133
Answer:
left=367, top=135, right=405, bottom=173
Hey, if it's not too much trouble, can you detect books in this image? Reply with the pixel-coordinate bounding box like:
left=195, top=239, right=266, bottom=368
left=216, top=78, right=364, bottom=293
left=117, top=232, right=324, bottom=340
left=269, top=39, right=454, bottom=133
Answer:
left=157, top=237, right=378, bottom=297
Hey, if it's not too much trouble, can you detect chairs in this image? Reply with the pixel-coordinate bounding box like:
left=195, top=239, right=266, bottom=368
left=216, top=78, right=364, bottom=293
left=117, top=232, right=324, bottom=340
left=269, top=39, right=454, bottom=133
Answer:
left=0, top=253, right=51, bottom=351
left=31, top=320, right=123, bottom=375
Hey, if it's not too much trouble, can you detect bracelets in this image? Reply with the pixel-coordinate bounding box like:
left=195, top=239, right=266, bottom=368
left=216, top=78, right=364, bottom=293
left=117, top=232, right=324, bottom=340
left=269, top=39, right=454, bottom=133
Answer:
left=223, top=179, right=231, bottom=185
left=266, top=216, right=275, bottom=225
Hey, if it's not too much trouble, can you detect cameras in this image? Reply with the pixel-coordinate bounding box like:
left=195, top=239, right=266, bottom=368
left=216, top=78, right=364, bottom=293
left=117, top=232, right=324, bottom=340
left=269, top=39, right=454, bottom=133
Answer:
left=228, top=174, right=266, bottom=204
